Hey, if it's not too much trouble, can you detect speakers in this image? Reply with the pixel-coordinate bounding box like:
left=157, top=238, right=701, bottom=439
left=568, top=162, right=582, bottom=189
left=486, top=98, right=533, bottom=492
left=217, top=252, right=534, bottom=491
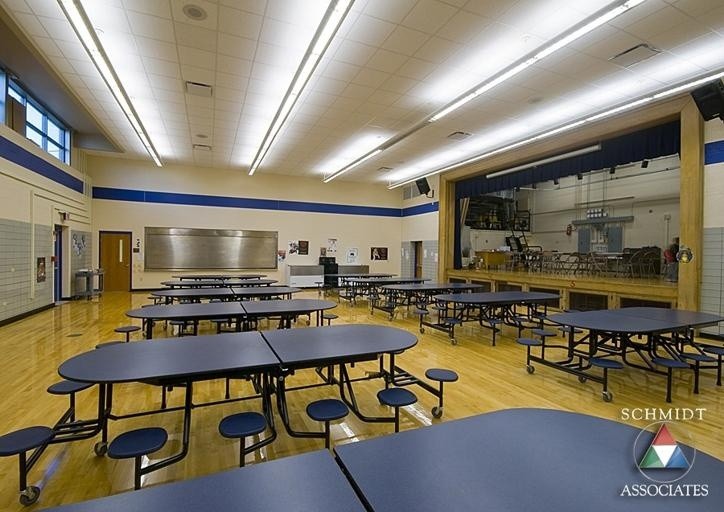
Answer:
left=416, top=178, right=431, bottom=195
left=690, top=79, right=723, bottom=121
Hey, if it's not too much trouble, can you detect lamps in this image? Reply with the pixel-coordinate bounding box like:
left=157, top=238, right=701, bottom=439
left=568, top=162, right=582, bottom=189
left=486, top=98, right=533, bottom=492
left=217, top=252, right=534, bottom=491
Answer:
left=56, top=0, right=165, bottom=169
left=245, top=0, right=355, bottom=176
left=322, top=0, right=658, bottom=184
left=385, top=65, right=724, bottom=193
